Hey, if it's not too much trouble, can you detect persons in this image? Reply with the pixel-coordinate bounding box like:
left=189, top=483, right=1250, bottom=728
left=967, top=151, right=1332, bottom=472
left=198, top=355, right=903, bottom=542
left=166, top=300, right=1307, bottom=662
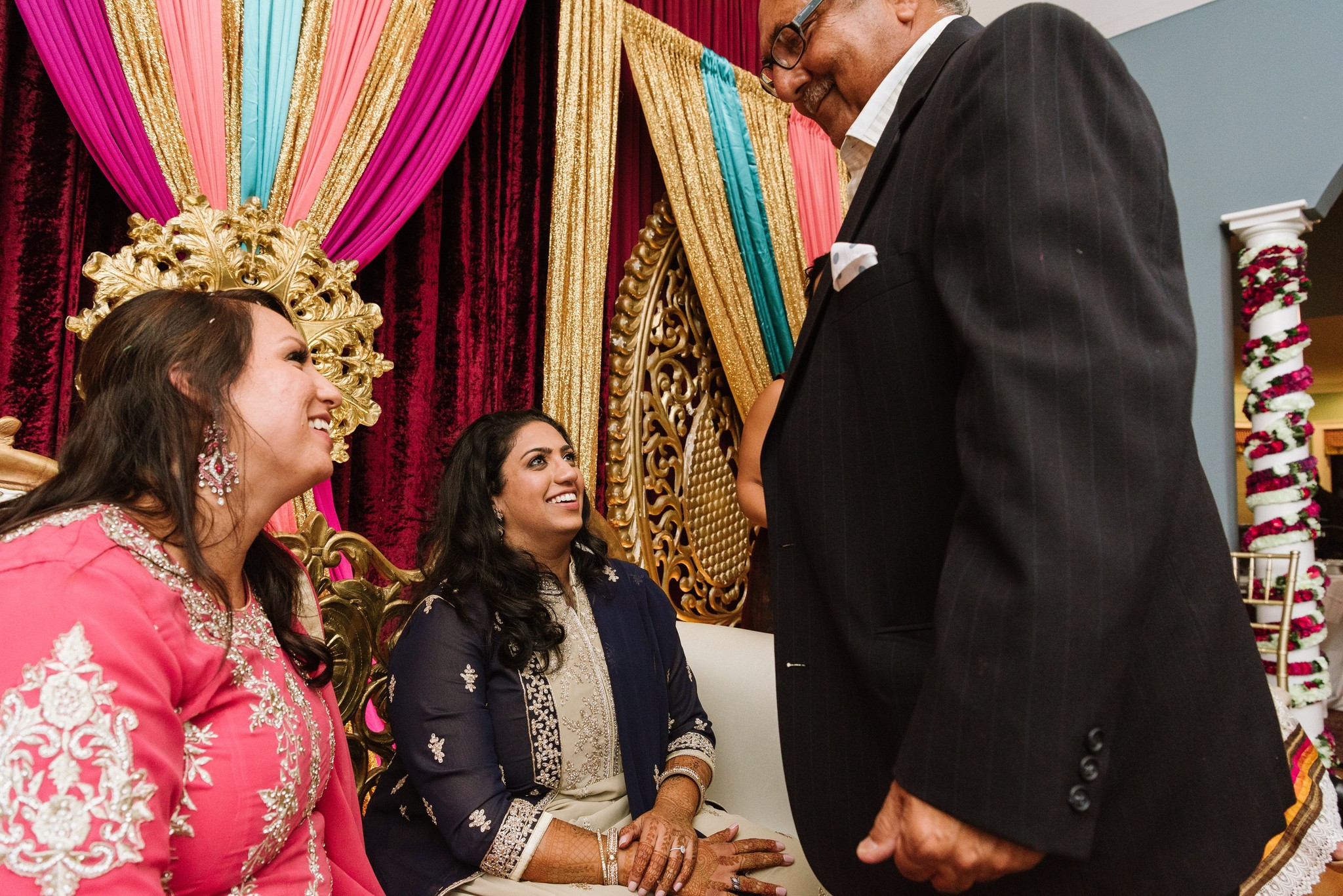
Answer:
left=733, top=253, right=832, bottom=633
left=758, top=1, right=1299, bottom=896
left=1, top=288, right=392, bottom=896
left=355, top=409, right=796, bottom=895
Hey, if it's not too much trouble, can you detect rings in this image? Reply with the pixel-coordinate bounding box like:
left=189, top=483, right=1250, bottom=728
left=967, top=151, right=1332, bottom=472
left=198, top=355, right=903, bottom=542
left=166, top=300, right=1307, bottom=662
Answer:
left=668, top=845, right=686, bottom=854
left=731, top=874, right=741, bottom=892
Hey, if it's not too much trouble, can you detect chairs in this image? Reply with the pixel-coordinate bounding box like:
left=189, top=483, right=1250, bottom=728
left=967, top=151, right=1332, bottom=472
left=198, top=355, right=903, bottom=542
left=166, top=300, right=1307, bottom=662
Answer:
left=1230, top=551, right=1302, bottom=708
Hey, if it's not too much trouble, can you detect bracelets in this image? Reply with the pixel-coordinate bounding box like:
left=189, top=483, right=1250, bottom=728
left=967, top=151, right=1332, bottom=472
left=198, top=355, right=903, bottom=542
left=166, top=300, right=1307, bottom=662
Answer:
left=659, top=767, right=706, bottom=817
left=596, top=828, right=608, bottom=886
left=606, top=827, right=619, bottom=885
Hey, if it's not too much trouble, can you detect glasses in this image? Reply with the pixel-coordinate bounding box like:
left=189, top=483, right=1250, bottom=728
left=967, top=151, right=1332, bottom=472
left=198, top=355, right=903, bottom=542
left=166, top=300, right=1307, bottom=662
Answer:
left=758, top=0, right=822, bottom=98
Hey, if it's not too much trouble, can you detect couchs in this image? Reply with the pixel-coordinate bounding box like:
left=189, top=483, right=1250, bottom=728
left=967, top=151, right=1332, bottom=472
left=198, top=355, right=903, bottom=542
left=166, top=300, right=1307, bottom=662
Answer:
left=0, top=416, right=800, bottom=845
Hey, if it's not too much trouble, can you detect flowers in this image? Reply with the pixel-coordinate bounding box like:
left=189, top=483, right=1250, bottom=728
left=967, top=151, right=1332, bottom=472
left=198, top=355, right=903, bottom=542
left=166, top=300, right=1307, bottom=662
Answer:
left=1237, top=240, right=1341, bottom=777
